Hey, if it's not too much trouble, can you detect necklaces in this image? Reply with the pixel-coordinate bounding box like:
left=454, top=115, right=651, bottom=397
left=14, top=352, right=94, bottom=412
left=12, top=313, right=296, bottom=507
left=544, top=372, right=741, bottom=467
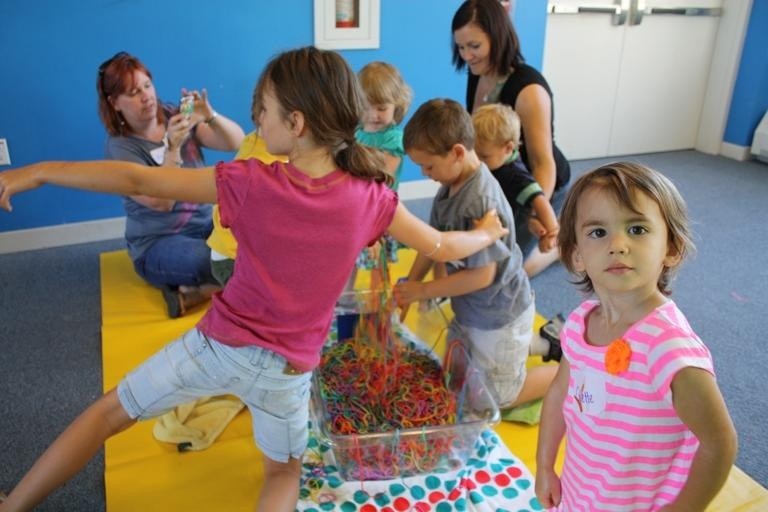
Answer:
left=481, top=91, right=493, bottom=103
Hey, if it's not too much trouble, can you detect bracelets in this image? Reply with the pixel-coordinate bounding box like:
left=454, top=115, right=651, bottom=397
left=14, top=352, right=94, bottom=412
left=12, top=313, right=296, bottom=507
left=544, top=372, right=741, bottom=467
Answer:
left=161, top=152, right=185, bottom=166
left=203, top=108, right=219, bottom=125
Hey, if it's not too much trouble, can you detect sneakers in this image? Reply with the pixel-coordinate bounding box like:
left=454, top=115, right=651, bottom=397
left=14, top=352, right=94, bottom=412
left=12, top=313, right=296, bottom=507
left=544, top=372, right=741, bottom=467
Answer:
left=539, top=314, right=564, bottom=362
left=162, top=285, right=188, bottom=320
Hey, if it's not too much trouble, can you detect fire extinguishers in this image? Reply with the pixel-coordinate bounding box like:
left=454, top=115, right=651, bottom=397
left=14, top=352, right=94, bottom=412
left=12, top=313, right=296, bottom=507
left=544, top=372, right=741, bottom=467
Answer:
left=335, top=0, right=355, bottom=28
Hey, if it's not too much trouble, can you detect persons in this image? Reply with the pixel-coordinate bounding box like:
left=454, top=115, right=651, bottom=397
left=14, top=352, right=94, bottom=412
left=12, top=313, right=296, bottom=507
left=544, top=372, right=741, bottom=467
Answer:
left=204, top=127, right=290, bottom=288
left=385, top=98, right=567, bottom=415
left=0, top=46, right=511, bottom=511
left=95, top=50, right=245, bottom=319
left=450, top=0, right=572, bottom=278
left=336, top=59, right=416, bottom=307
left=529, top=157, right=738, bottom=512
left=471, top=101, right=560, bottom=253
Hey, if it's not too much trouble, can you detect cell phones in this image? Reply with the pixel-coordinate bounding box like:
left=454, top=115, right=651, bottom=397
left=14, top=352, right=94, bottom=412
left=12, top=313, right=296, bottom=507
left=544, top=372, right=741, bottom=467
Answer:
left=177, top=94, right=196, bottom=118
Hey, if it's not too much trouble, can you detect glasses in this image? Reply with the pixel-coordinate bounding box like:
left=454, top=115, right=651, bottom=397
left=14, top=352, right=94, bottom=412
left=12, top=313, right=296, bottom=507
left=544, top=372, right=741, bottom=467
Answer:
left=98, top=51, right=131, bottom=97
